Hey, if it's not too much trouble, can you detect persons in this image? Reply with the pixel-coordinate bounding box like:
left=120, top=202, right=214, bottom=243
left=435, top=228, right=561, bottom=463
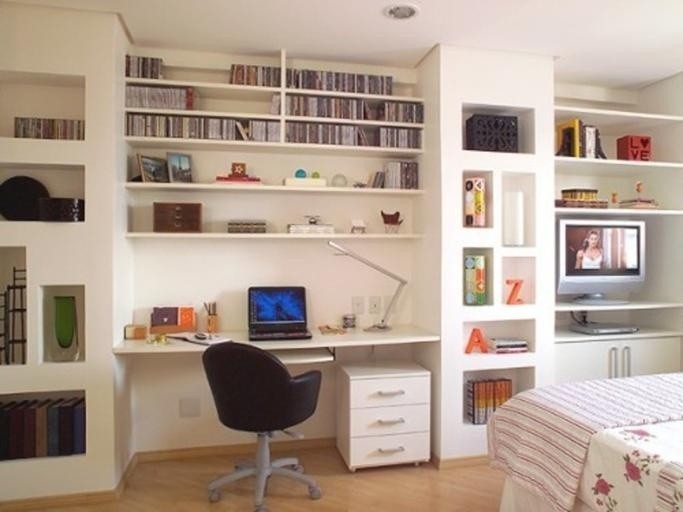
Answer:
left=575, top=231, right=605, bottom=270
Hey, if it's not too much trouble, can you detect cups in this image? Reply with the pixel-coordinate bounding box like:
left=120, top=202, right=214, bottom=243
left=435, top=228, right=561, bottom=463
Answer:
left=342, top=314, right=355, bottom=331
left=206, top=314, right=220, bottom=333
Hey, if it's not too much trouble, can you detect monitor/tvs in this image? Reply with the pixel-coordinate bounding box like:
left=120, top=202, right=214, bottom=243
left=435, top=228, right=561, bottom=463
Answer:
left=557, top=219, right=646, bottom=305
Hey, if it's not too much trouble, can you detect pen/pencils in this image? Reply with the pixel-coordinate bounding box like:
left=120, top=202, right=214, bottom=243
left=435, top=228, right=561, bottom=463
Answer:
left=205, top=303, right=218, bottom=316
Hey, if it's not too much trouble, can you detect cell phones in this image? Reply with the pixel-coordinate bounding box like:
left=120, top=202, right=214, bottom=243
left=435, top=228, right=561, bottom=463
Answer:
left=196, top=333, right=206, bottom=339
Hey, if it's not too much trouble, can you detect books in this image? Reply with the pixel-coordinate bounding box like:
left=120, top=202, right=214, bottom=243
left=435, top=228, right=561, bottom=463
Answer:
left=126, top=114, right=280, bottom=141
left=367, top=162, right=420, bottom=188
left=286, top=97, right=422, bottom=123
left=270, top=95, right=280, bottom=115
left=464, top=378, right=513, bottom=424
left=0, top=398, right=86, bottom=459
left=286, top=68, right=392, bottom=95
left=229, top=63, right=281, bottom=87
left=125, top=86, right=199, bottom=110
left=491, top=337, right=528, bottom=353
left=125, top=55, right=164, bottom=79
left=286, top=121, right=421, bottom=149
left=13, top=116, right=84, bottom=140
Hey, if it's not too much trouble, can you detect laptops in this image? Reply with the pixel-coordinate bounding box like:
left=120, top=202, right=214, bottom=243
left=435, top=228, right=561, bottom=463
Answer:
left=248, top=287, right=312, bottom=340
